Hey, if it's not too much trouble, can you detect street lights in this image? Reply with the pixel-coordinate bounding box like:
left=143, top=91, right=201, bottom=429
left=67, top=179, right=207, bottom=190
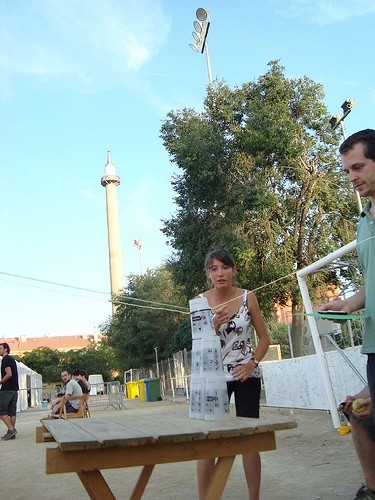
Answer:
left=190, top=8, right=218, bottom=119
left=328, top=98, right=363, bottom=217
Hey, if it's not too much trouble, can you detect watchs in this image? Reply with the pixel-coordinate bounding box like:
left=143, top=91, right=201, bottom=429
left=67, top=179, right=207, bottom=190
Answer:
left=250, top=357, right=259, bottom=367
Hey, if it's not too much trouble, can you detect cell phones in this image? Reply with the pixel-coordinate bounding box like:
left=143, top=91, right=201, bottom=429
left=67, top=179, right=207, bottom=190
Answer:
left=318, top=310, right=348, bottom=315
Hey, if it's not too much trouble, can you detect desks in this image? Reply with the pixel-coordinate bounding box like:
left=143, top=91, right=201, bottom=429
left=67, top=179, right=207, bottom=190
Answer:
left=35, top=407, right=299, bottom=500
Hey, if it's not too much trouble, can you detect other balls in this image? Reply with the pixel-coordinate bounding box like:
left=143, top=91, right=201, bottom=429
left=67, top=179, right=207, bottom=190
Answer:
left=353, top=398, right=367, bottom=413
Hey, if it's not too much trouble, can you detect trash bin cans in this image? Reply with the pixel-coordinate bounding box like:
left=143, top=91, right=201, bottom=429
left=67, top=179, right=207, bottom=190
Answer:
left=126, top=381, right=138, bottom=398
left=143, top=379, right=162, bottom=400
left=139, top=379, right=146, bottom=401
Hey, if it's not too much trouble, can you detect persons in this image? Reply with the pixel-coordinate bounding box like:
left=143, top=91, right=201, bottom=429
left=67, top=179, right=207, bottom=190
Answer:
left=337, top=385, right=375, bottom=500
left=71, top=370, right=91, bottom=393
left=0, top=342, right=19, bottom=440
left=193, top=249, right=271, bottom=499
left=50, top=370, right=83, bottom=420
left=319, top=129, right=375, bottom=417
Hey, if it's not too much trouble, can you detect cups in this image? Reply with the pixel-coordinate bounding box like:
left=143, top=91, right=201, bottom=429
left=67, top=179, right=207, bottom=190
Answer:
left=189, top=297, right=231, bottom=421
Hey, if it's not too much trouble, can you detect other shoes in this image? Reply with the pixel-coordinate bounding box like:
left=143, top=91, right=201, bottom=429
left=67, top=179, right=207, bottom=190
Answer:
left=1, top=434, right=15, bottom=439
left=4, top=428, right=17, bottom=439
left=354, top=482, right=375, bottom=500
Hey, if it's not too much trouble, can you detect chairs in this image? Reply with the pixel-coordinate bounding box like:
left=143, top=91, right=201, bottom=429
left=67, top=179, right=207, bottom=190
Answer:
left=50, top=392, right=92, bottom=419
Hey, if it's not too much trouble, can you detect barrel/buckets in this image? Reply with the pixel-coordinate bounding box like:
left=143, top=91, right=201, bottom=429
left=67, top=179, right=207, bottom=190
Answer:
left=42, top=400, right=48, bottom=406
